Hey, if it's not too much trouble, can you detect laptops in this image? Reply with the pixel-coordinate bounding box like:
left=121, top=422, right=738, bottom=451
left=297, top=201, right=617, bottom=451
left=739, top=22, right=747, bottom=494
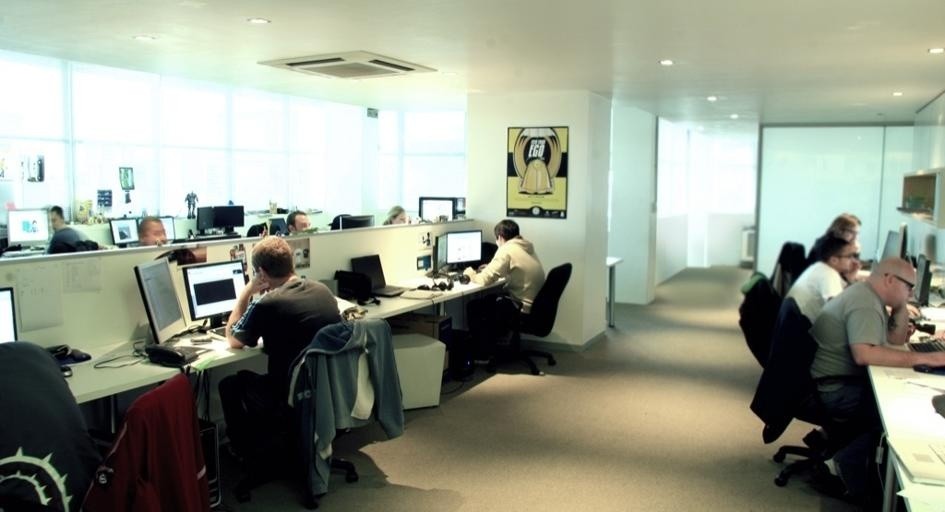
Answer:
left=350, top=254, right=409, bottom=298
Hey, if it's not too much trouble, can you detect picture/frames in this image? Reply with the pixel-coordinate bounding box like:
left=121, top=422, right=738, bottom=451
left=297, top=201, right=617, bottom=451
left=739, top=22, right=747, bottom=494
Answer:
left=506, top=125, right=569, bottom=219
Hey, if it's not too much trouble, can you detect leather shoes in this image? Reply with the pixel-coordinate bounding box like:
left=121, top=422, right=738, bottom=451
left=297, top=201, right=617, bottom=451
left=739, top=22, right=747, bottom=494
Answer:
left=810, top=461, right=846, bottom=495
left=803, top=429, right=827, bottom=451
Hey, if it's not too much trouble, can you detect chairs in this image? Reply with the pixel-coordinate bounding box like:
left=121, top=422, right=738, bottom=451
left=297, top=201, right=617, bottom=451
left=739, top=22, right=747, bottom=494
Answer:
left=738, top=278, right=783, bottom=369
left=769, top=241, right=807, bottom=297
left=486, top=263, right=572, bottom=375
left=750, top=298, right=853, bottom=487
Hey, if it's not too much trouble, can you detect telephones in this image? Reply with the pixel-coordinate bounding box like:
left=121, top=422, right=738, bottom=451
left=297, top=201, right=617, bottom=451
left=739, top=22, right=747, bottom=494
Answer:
left=146, top=346, right=198, bottom=367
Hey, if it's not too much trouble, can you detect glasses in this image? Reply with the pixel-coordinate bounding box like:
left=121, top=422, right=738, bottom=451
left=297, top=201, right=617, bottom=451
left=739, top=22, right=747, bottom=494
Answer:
left=884, top=272, right=916, bottom=293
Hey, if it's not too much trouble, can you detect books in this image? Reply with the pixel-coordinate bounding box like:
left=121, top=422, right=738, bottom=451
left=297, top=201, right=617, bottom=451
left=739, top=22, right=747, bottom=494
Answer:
left=400, top=289, right=444, bottom=300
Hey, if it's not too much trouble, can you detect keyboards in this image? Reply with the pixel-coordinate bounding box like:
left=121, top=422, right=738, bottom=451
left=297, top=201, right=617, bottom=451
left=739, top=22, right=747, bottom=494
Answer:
left=909, top=341, right=945, bottom=373
left=207, top=327, right=227, bottom=341
left=5, top=250, right=46, bottom=258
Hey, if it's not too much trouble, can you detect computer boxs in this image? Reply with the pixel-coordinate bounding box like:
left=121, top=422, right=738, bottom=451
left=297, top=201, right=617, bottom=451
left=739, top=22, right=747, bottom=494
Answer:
left=398, top=313, right=452, bottom=370
left=198, top=417, right=222, bottom=508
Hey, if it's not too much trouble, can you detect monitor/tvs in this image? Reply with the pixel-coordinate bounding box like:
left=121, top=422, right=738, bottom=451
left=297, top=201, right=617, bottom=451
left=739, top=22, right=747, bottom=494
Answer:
left=182, top=259, right=246, bottom=321
left=338, top=214, right=375, bottom=230
left=136, top=216, right=176, bottom=241
left=914, top=253, right=932, bottom=307
left=197, top=206, right=215, bottom=230
left=445, top=230, right=482, bottom=264
left=134, top=257, right=187, bottom=344
left=0, top=287, right=17, bottom=343
left=898, top=223, right=908, bottom=262
left=213, top=207, right=245, bottom=228
left=433, top=233, right=447, bottom=271
left=5, top=207, right=52, bottom=246
left=418, top=197, right=456, bottom=221
left=109, top=216, right=140, bottom=244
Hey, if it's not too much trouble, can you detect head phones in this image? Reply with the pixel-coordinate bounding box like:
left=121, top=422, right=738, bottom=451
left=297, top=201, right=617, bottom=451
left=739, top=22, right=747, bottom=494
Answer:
left=432, top=272, right=454, bottom=291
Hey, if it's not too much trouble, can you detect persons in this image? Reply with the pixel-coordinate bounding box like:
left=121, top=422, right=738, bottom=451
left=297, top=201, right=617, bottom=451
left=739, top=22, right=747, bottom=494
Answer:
left=773, top=238, right=867, bottom=334
left=805, top=212, right=863, bottom=260
left=1, top=343, right=93, bottom=511
left=807, top=256, right=944, bottom=499
left=382, top=205, right=407, bottom=225
left=46, top=206, right=82, bottom=254
left=218, top=234, right=343, bottom=413
left=286, top=210, right=312, bottom=233
left=461, top=217, right=546, bottom=366
left=139, top=216, right=168, bottom=245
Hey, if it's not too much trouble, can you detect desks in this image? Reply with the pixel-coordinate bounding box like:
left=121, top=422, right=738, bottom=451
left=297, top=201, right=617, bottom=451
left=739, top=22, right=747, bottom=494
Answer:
left=856, top=260, right=945, bottom=512
left=56, top=267, right=505, bottom=509
left=607, top=256, right=624, bottom=328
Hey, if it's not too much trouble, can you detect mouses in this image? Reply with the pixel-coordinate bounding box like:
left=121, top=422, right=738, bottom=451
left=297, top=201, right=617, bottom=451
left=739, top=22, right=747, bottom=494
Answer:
left=914, top=364, right=934, bottom=373
left=60, top=364, right=74, bottom=378
